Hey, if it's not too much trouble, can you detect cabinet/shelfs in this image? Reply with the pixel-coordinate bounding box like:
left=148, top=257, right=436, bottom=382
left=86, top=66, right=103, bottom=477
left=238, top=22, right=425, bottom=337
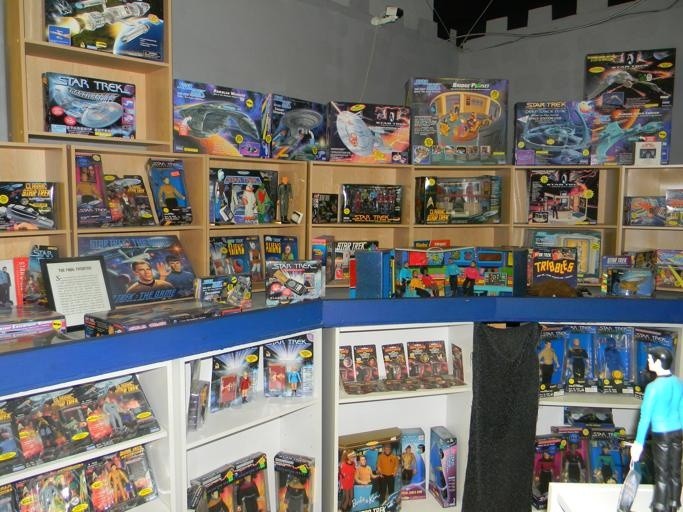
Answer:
left=308, top=159, right=411, bottom=287
left=1, top=0, right=174, bottom=154
left=1, top=350, right=175, bottom=512
left=175, top=319, right=322, bottom=511
left=512, top=165, right=622, bottom=286
left=206, top=154, right=307, bottom=293
left=620, top=164, right=683, bottom=291
left=413, top=165, right=510, bottom=246
left=68, top=145, right=208, bottom=309
left=324, top=298, right=474, bottom=512
left=0, top=141, right=70, bottom=309
left=530, top=298, right=683, bottom=512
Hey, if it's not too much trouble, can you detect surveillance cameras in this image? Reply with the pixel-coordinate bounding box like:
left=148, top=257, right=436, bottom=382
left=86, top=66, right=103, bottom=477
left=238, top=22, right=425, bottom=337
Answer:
left=372, top=5, right=406, bottom=27
